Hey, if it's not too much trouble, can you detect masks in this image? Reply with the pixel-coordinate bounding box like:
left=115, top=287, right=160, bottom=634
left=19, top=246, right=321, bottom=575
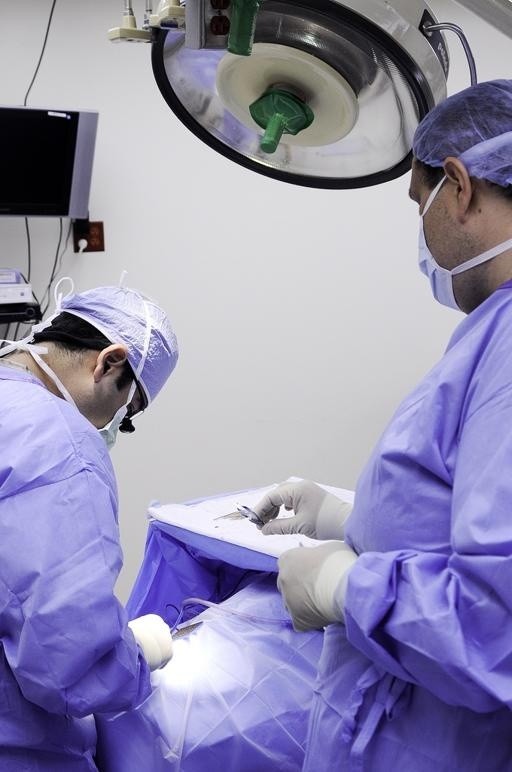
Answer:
left=97, top=407, right=128, bottom=450
left=418, top=215, right=461, bottom=310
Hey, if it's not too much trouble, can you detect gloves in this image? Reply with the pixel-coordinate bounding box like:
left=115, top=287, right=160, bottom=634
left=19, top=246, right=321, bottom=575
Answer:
left=128, top=614, right=173, bottom=672
left=251, top=480, right=353, bottom=540
left=277, top=542, right=358, bottom=631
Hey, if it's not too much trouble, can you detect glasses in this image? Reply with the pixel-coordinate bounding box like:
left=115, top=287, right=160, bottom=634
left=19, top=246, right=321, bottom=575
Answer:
left=121, top=359, right=145, bottom=421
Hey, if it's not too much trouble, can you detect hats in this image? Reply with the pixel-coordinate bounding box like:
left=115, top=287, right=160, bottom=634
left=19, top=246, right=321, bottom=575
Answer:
left=63, top=287, right=179, bottom=404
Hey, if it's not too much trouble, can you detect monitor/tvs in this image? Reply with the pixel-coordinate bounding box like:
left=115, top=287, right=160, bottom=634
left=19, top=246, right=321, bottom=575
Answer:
left=0, top=108, right=101, bottom=219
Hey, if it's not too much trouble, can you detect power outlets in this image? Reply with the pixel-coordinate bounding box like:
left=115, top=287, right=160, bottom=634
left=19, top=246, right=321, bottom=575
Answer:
left=72, top=221, right=108, bottom=255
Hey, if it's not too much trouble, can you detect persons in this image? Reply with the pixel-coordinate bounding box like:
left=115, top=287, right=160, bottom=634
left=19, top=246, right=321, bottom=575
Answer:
left=252, top=77, right=512, bottom=772
left=0, top=285, right=180, bottom=772
